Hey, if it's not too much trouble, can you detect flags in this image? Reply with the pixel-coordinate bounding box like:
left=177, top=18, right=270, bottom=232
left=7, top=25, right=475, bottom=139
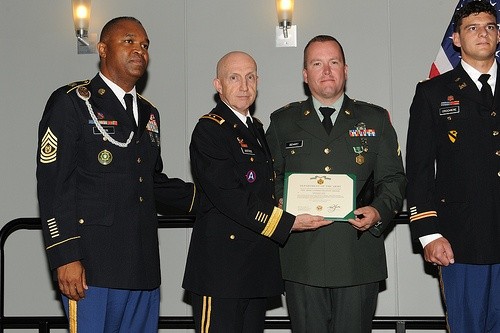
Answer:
left=428, top=0, right=500, bottom=79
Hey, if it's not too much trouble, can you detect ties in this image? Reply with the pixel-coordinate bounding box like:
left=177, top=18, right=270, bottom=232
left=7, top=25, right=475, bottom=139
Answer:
left=318, top=106, right=337, bottom=135
left=476, top=74, right=494, bottom=98
left=123, top=93, right=137, bottom=129
left=245, top=116, right=259, bottom=142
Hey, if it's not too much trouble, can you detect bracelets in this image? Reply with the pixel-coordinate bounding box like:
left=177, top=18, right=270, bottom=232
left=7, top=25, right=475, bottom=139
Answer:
left=375, top=221, right=382, bottom=228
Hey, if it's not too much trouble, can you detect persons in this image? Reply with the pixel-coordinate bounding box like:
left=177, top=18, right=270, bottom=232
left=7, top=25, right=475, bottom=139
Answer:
left=36, top=17, right=198, bottom=333
left=183, top=51, right=334, bottom=333
left=265, top=36, right=405, bottom=333
left=406, top=0, right=500, bottom=333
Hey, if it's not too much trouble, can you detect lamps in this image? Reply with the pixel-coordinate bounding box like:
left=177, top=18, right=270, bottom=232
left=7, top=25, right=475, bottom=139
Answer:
left=72, top=0, right=98, bottom=54
left=275, top=0, right=297, bottom=48
